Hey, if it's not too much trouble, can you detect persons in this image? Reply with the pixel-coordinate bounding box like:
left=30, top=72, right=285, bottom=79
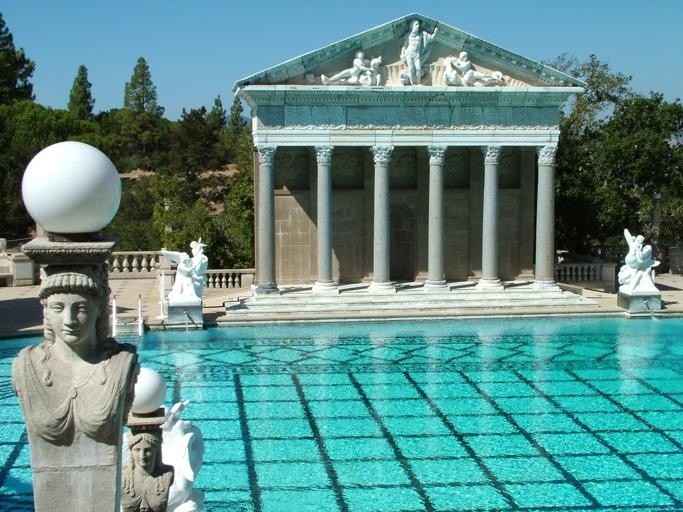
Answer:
left=443, top=51, right=503, bottom=86
left=617, top=244, right=657, bottom=293
left=339, top=55, right=385, bottom=86
left=172, top=252, right=205, bottom=303
left=319, top=51, right=372, bottom=85
left=618, top=228, right=645, bottom=269
left=400, top=19, right=439, bottom=85
left=11, top=264, right=139, bottom=512
left=119, top=429, right=174, bottom=512
left=162, top=235, right=209, bottom=294
